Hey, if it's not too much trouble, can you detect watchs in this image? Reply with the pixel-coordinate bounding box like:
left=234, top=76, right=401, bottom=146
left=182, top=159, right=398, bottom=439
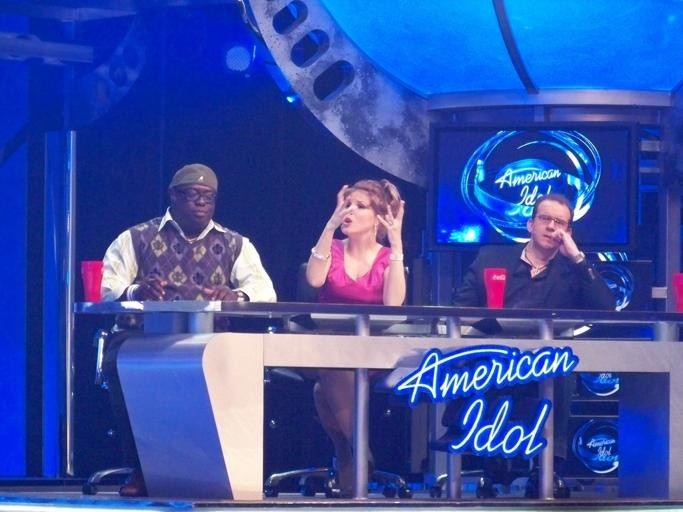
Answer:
left=570, top=251, right=586, bottom=265
left=232, top=289, right=246, bottom=303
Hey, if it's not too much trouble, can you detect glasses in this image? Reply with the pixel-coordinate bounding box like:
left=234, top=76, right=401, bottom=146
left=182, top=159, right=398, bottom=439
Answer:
left=537, top=213, right=569, bottom=228
left=174, top=188, right=216, bottom=204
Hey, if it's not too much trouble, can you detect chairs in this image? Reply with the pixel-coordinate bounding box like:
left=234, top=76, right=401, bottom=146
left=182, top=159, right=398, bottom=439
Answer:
left=80, top=258, right=146, bottom=496
left=430, top=266, right=574, bottom=501
left=668, top=269, right=682, bottom=312
left=263, top=262, right=414, bottom=498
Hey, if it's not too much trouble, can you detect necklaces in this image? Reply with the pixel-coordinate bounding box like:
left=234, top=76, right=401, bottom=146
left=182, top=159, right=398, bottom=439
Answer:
left=525, top=248, right=552, bottom=275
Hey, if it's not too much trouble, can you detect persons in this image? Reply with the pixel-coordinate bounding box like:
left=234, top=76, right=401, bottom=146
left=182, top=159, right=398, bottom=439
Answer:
left=126, top=284, right=138, bottom=301
left=98, top=162, right=279, bottom=499
left=427, top=193, right=616, bottom=502
left=306, top=178, right=408, bottom=497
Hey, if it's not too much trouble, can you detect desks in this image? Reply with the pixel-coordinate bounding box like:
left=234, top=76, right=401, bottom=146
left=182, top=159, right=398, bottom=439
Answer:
left=72, top=298, right=681, bottom=504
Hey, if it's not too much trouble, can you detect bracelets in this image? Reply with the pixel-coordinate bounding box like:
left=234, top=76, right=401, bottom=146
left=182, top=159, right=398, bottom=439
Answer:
left=311, top=246, right=333, bottom=261
left=388, top=254, right=404, bottom=261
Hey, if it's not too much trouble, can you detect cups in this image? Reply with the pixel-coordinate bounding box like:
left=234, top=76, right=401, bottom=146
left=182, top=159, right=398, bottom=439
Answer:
left=80, top=260, right=104, bottom=302
left=483, top=267, right=508, bottom=309
left=672, top=272, right=683, bottom=314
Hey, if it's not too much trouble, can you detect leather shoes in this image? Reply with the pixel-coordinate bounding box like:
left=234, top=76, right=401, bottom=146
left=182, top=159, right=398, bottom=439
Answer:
left=478, top=476, right=497, bottom=496
left=526, top=479, right=558, bottom=496
left=119, top=466, right=147, bottom=496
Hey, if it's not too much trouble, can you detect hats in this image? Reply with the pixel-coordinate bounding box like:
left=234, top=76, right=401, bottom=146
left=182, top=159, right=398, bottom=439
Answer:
left=169, top=163, right=218, bottom=190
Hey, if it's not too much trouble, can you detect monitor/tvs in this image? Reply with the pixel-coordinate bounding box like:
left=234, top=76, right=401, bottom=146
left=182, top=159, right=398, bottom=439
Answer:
left=426, top=114, right=641, bottom=254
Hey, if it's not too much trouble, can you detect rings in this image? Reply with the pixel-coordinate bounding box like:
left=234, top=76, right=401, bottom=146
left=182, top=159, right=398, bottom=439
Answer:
left=145, top=284, right=151, bottom=290
